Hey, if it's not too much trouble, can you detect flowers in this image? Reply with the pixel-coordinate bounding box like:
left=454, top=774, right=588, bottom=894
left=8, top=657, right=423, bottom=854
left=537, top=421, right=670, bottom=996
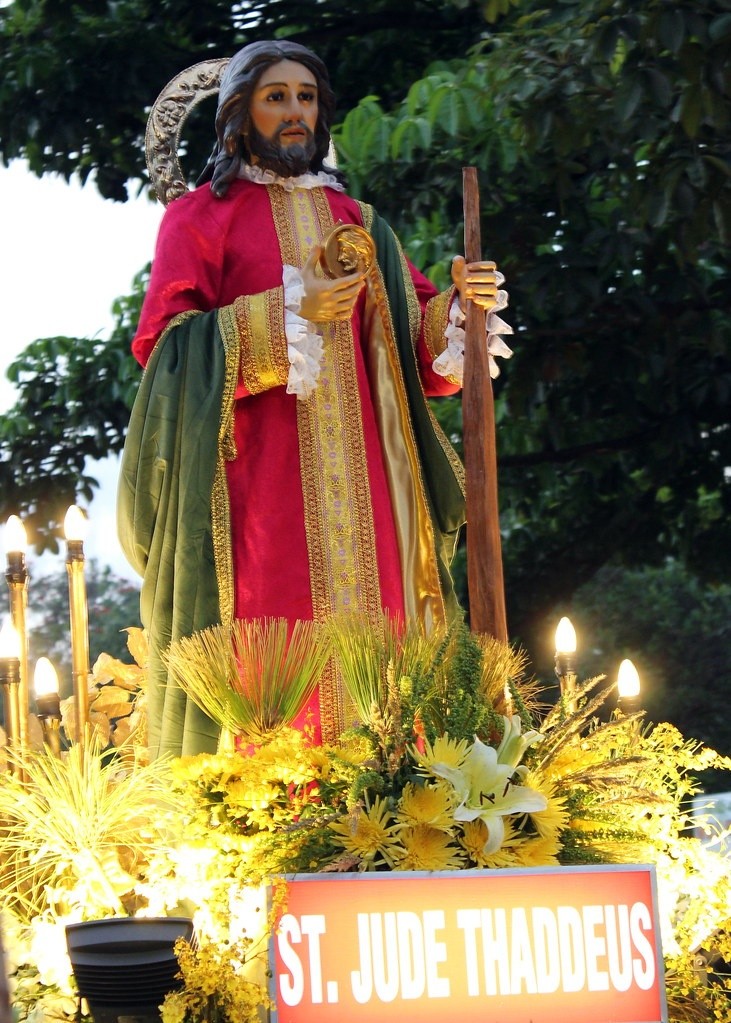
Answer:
left=0, top=606, right=731, bottom=1023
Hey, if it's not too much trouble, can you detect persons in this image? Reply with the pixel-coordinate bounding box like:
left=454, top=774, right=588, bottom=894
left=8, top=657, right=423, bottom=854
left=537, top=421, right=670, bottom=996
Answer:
left=112, top=43, right=516, bottom=823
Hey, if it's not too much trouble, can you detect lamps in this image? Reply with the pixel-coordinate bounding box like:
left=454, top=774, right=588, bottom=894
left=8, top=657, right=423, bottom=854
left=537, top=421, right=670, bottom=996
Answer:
left=64, top=916, right=200, bottom=1023
